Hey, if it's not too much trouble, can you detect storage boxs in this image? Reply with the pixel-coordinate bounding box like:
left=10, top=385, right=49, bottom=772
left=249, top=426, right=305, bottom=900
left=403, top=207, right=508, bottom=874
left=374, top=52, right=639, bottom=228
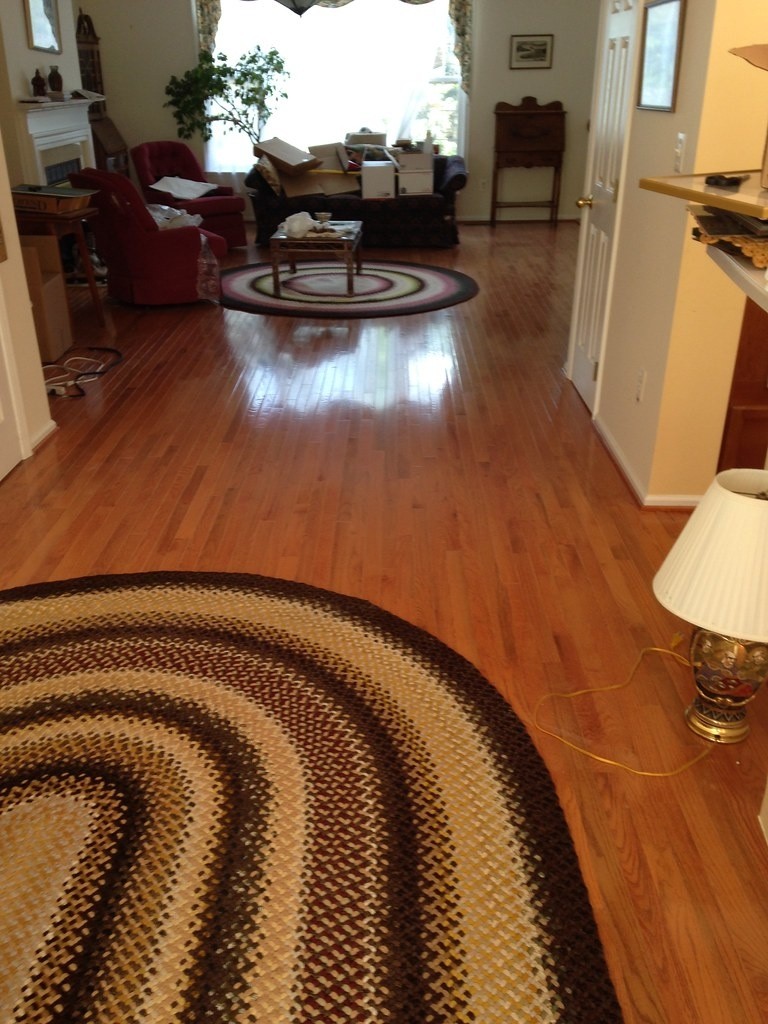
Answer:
left=362, top=161, right=395, bottom=200
left=254, top=137, right=323, bottom=178
left=729, top=44, right=768, bottom=190
left=12, top=193, right=91, bottom=215
left=384, top=130, right=434, bottom=196
left=19, top=235, right=73, bottom=363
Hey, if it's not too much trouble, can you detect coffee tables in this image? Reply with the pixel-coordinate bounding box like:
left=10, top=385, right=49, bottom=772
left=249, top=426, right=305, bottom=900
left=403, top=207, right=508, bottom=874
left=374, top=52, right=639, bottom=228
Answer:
left=269, top=220, right=362, bottom=299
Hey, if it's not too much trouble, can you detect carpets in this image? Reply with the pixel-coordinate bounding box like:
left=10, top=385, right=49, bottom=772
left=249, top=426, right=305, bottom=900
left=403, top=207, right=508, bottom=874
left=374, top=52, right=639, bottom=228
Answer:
left=220, top=259, right=479, bottom=319
left=0, top=571, right=624, bottom=1024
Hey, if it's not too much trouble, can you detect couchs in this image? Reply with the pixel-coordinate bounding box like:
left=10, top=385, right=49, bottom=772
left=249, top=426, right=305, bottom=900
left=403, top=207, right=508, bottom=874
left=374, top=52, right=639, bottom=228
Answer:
left=244, top=154, right=468, bottom=250
left=70, top=167, right=225, bottom=308
left=130, top=141, right=247, bottom=254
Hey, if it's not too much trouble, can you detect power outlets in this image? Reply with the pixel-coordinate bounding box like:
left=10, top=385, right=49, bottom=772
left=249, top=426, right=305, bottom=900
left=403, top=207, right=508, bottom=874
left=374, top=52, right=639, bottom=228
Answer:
left=633, top=365, right=648, bottom=404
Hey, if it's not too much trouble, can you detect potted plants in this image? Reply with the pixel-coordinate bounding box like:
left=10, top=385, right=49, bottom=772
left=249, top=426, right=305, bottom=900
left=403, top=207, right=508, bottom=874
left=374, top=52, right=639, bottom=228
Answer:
left=162, top=45, right=290, bottom=244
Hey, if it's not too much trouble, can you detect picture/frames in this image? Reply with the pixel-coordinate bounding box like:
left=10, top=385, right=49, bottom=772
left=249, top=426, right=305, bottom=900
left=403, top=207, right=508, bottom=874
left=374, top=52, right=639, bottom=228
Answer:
left=633, top=1, right=686, bottom=114
left=639, top=169, right=768, bottom=222
left=24, top=0, right=62, bottom=55
left=510, top=34, right=553, bottom=70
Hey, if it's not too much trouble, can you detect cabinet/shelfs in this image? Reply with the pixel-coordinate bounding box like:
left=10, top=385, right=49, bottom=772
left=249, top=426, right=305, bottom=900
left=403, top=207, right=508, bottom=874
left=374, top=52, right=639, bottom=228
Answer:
left=705, top=244, right=768, bottom=478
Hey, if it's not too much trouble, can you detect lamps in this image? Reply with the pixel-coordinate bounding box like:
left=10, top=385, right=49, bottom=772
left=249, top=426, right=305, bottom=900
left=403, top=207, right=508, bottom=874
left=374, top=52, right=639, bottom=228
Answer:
left=653, top=468, right=768, bottom=745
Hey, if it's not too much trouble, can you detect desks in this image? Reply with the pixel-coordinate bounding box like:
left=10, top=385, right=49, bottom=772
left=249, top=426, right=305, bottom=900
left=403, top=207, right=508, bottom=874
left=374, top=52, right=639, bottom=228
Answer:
left=15, top=206, right=117, bottom=339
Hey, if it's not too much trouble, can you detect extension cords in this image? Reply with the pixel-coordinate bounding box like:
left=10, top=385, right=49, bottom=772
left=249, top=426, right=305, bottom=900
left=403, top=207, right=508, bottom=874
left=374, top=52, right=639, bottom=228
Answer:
left=45, top=384, right=65, bottom=395
